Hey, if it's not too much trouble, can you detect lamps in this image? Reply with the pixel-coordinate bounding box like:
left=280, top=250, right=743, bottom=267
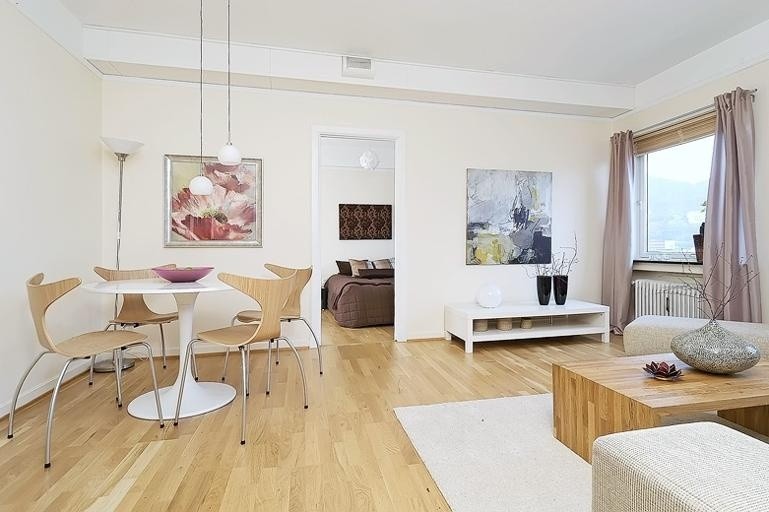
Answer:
left=188, top=0, right=213, bottom=196
left=218, top=1, right=241, bottom=165
left=361, top=145, right=379, bottom=170
left=93, top=136, right=144, bottom=373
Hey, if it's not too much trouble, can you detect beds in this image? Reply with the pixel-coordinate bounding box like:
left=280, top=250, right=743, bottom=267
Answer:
left=324, top=258, right=394, bottom=328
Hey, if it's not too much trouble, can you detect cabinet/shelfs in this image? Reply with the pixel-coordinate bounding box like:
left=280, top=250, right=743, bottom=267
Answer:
left=444, top=299, right=610, bottom=353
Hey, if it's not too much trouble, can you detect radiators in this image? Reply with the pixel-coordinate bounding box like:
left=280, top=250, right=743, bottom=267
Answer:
left=632, top=279, right=705, bottom=318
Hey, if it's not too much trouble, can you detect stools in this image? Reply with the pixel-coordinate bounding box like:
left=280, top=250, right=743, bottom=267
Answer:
left=591, top=422, right=768, bottom=512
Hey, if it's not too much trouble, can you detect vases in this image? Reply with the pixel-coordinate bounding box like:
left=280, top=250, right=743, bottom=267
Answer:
left=668, top=319, right=760, bottom=375
left=536, top=275, right=569, bottom=305
left=693, top=235, right=704, bottom=262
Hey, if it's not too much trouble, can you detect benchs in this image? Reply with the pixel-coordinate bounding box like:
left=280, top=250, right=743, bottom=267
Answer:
left=623, top=315, right=769, bottom=356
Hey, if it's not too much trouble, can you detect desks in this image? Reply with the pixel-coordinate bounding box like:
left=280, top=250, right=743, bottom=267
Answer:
left=79, top=275, right=238, bottom=423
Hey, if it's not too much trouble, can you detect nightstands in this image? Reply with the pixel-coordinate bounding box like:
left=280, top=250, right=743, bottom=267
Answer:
left=321, top=288, right=328, bottom=309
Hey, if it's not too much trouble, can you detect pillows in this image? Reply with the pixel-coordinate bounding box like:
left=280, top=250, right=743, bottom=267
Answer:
left=335, top=259, right=394, bottom=278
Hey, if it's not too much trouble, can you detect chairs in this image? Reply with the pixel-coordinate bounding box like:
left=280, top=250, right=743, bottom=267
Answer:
left=93, top=263, right=202, bottom=382
left=170, top=268, right=310, bottom=446
left=219, top=264, right=325, bottom=387
left=7, top=272, right=166, bottom=469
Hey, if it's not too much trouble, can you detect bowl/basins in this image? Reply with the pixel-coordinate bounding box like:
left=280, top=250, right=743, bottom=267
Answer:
left=153, top=268, right=212, bottom=282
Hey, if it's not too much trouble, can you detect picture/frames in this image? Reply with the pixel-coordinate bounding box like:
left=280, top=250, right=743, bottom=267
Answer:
left=164, top=154, right=262, bottom=247
left=339, top=204, right=392, bottom=239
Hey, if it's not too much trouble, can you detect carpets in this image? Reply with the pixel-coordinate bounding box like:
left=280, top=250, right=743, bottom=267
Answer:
left=393, top=394, right=591, bottom=512
